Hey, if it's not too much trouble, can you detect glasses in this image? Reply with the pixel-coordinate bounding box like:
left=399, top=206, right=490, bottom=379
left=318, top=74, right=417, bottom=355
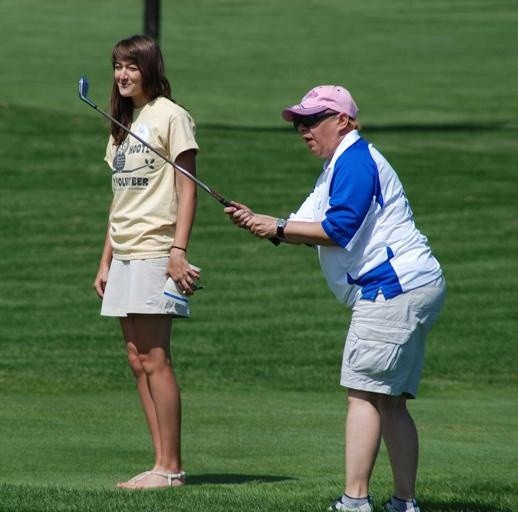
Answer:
left=294, top=112, right=338, bottom=128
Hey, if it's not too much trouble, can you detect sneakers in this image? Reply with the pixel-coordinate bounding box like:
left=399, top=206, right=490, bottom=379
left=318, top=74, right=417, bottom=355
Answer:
left=326, top=497, right=374, bottom=512
left=381, top=501, right=420, bottom=512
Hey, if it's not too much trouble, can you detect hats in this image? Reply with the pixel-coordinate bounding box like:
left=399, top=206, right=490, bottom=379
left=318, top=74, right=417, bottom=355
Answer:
left=283, top=85, right=358, bottom=121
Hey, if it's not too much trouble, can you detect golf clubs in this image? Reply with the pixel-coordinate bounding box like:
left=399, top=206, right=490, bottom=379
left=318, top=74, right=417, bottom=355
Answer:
left=78, top=77, right=281, bottom=246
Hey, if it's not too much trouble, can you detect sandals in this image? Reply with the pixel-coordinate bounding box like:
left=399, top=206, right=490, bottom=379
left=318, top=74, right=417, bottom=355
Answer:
left=117, top=470, right=186, bottom=490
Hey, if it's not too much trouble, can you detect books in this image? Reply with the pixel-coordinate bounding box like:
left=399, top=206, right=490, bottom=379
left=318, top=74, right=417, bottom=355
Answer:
left=160, top=263, right=201, bottom=303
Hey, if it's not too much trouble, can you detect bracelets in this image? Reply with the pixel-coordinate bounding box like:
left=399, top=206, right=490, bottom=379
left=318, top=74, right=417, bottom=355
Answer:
left=170, top=245, right=186, bottom=252
left=275, top=218, right=286, bottom=241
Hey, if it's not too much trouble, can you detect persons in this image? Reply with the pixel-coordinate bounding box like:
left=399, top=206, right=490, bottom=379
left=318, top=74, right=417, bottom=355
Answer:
left=225, top=82, right=446, bottom=511
left=89, top=34, right=201, bottom=493
